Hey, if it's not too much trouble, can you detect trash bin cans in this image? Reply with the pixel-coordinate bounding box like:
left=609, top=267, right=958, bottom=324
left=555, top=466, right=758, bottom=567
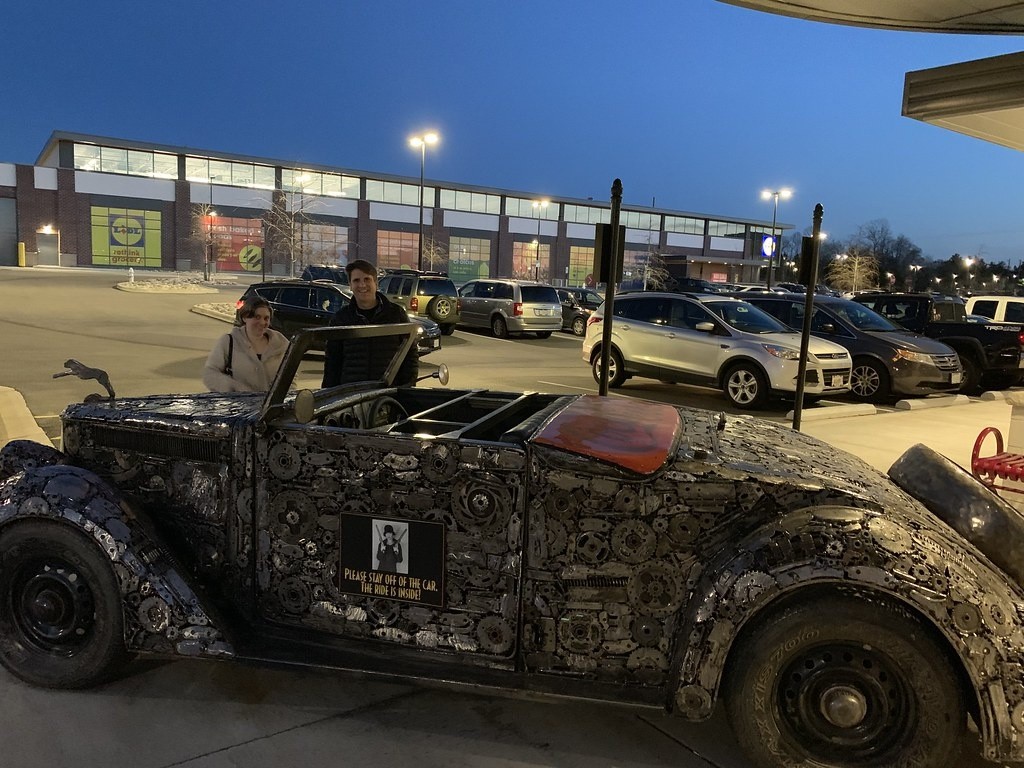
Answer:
left=208, top=261, right=216, bottom=273
left=25, top=252, right=38, bottom=267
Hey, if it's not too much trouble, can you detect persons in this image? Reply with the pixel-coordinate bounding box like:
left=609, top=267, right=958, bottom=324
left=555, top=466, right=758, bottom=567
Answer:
left=202, top=296, right=298, bottom=393
left=320, top=260, right=420, bottom=387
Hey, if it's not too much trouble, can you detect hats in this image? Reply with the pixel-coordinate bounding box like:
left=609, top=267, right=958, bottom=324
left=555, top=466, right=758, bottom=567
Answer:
left=383, top=525, right=396, bottom=536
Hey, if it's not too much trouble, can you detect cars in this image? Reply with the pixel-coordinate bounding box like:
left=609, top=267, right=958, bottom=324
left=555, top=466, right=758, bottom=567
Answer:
left=552, top=286, right=605, bottom=338
left=0, top=322, right=1024, bottom=768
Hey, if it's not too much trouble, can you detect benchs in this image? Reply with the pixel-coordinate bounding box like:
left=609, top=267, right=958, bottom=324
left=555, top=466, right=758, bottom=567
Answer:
left=970, top=425, right=1024, bottom=495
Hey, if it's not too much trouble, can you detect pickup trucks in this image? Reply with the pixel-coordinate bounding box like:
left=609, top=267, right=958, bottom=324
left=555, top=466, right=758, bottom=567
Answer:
left=848, top=292, right=1024, bottom=396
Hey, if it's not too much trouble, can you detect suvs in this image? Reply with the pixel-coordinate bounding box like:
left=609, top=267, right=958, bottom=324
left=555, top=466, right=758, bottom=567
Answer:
left=582, top=290, right=853, bottom=412
left=669, top=279, right=833, bottom=323
left=708, top=293, right=965, bottom=405
left=235, top=279, right=442, bottom=357
left=299, top=264, right=463, bottom=337
left=457, top=278, right=563, bottom=339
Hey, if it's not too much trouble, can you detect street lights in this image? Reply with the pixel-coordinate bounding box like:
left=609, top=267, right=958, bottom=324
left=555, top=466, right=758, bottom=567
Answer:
left=910, top=265, right=920, bottom=292
left=410, top=133, right=438, bottom=272
left=760, top=189, right=792, bottom=291
left=532, top=200, right=548, bottom=281
